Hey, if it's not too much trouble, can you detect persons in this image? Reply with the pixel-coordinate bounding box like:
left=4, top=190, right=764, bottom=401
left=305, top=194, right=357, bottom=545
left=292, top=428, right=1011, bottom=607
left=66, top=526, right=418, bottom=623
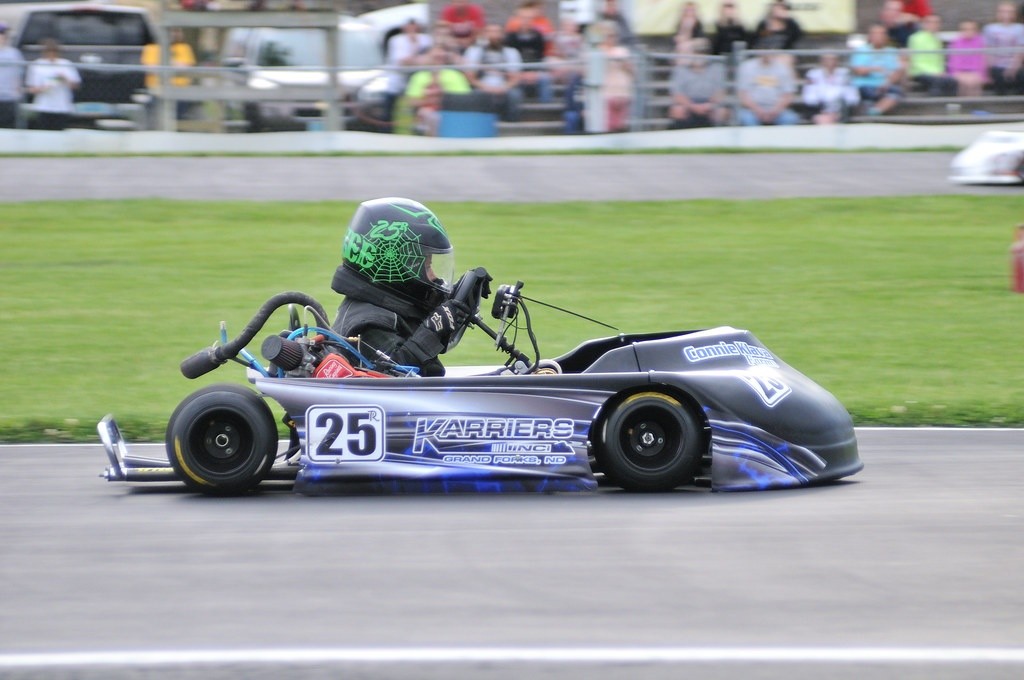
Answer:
left=504, top=0, right=552, bottom=102
left=575, top=21, right=637, bottom=135
left=331, top=197, right=494, bottom=378
left=0, top=23, right=25, bottom=128
left=669, top=3, right=703, bottom=45
left=141, top=26, right=196, bottom=120
left=907, top=12, right=957, bottom=98
left=710, top=0, right=747, bottom=55
left=388, top=0, right=521, bottom=137
left=848, top=21, right=911, bottom=115
left=21, top=37, right=82, bottom=113
left=982, top=0, right=1024, bottom=95
left=732, top=36, right=800, bottom=124
left=598, top=0, right=630, bottom=36
left=787, top=54, right=861, bottom=124
left=947, top=19, right=992, bottom=94
left=667, top=37, right=727, bottom=130
left=880, top=0, right=933, bottom=48
left=750, top=0, right=802, bottom=50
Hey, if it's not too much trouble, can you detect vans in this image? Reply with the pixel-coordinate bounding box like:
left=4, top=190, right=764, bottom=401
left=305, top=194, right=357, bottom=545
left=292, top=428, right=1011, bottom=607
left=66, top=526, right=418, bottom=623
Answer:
left=6, top=7, right=170, bottom=131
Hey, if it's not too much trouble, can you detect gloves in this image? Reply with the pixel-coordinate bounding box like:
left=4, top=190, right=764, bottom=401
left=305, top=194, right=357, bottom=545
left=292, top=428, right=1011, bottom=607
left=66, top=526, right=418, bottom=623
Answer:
left=426, top=299, right=470, bottom=333
left=463, top=267, right=493, bottom=299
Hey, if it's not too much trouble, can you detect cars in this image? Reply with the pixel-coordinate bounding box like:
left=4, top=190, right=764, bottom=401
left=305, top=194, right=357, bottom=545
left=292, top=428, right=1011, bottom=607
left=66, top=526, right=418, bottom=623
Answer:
left=217, top=14, right=404, bottom=132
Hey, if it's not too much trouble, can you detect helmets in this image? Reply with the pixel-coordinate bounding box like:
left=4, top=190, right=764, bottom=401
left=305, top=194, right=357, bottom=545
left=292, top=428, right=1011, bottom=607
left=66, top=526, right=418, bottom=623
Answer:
left=342, top=197, right=452, bottom=306
